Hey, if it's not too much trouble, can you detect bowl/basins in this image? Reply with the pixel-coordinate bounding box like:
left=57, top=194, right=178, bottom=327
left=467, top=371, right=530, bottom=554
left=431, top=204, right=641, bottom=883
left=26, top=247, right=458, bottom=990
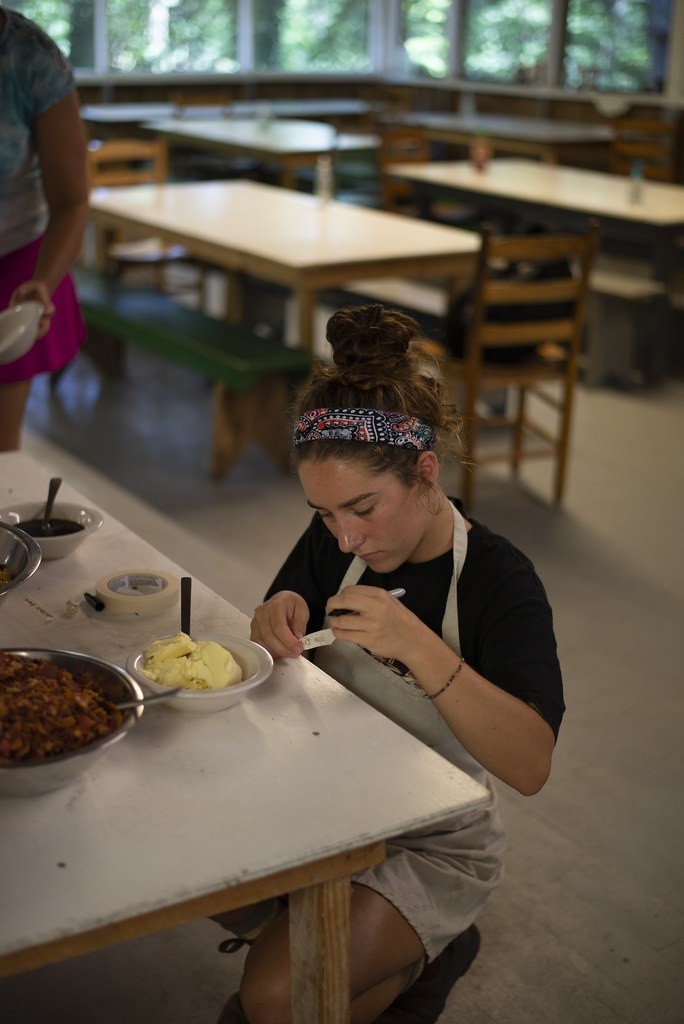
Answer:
left=0, top=522, right=42, bottom=603
left=0, top=302, right=47, bottom=363
left=126, top=632, right=274, bottom=713
left=0, top=648, right=144, bottom=799
left=0, top=498, right=103, bottom=560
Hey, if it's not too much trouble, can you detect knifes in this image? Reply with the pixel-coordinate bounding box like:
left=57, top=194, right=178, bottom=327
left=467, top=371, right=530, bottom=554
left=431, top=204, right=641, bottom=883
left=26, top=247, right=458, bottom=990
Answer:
left=298, top=588, right=407, bottom=650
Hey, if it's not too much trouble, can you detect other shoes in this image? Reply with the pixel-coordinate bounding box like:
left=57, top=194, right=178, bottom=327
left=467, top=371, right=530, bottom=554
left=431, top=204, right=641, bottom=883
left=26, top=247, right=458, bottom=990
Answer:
left=365, top=923, right=481, bottom=1024
left=216, top=937, right=248, bottom=1024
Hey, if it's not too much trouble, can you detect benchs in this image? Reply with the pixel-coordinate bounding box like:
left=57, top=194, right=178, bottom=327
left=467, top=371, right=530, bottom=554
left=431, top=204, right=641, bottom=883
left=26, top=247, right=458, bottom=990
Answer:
left=173, top=134, right=388, bottom=180
left=330, top=277, right=450, bottom=341
left=64, top=269, right=317, bottom=472
left=493, top=254, right=673, bottom=383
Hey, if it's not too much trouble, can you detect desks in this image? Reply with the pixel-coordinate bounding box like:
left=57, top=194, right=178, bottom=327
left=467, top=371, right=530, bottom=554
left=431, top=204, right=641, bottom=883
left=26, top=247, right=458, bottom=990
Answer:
left=381, top=153, right=684, bottom=384
left=394, top=112, right=614, bottom=168
left=89, top=182, right=486, bottom=469
left=140, top=121, right=390, bottom=196
left=0, top=438, right=494, bottom=1024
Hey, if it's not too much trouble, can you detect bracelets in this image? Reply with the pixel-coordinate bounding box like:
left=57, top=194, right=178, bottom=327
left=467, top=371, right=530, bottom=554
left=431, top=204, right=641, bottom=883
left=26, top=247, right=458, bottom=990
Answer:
left=422, top=657, right=465, bottom=699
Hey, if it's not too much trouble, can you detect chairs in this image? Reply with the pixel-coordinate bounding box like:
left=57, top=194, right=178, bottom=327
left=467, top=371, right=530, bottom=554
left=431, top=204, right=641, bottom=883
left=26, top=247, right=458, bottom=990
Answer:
left=608, top=117, right=675, bottom=183
left=87, top=135, right=210, bottom=357
left=419, top=226, right=594, bottom=510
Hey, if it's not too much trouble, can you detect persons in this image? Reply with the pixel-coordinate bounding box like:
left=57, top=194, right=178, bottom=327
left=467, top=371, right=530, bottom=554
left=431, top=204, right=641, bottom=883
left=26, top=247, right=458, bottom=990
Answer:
left=0, top=5, right=88, bottom=455
left=201, top=304, right=568, bottom=1024
